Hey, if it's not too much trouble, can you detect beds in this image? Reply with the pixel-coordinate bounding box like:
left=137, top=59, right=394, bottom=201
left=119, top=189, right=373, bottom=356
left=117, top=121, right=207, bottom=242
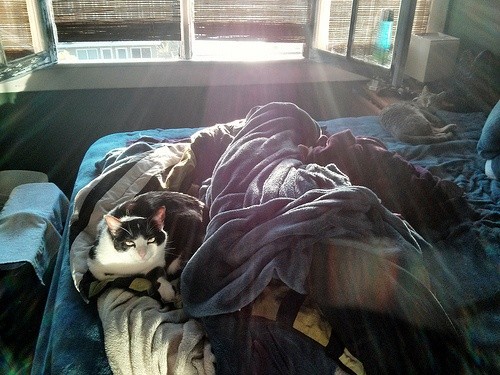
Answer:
left=34, top=103, right=500, bottom=375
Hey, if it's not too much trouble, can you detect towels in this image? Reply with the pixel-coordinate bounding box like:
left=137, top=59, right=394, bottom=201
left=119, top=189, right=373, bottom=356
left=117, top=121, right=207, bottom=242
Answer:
left=296, top=129, right=467, bottom=245
left=0, top=183, right=70, bottom=287
left=177, top=101, right=475, bottom=318
left=95, top=289, right=222, bottom=375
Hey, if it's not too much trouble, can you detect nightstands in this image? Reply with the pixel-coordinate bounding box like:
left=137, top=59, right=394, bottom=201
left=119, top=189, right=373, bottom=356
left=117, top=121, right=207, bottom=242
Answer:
left=349, top=84, right=411, bottom=115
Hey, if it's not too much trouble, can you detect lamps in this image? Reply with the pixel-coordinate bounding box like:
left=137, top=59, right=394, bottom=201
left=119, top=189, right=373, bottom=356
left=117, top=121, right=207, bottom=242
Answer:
left=405, top=32, right=460, bottom=96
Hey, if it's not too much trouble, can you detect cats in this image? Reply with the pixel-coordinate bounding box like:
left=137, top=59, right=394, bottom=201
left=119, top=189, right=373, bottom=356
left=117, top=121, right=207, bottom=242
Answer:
left=87, top=191, right=209, bottom=301
left=378, top=85, right=457, bottom=145
